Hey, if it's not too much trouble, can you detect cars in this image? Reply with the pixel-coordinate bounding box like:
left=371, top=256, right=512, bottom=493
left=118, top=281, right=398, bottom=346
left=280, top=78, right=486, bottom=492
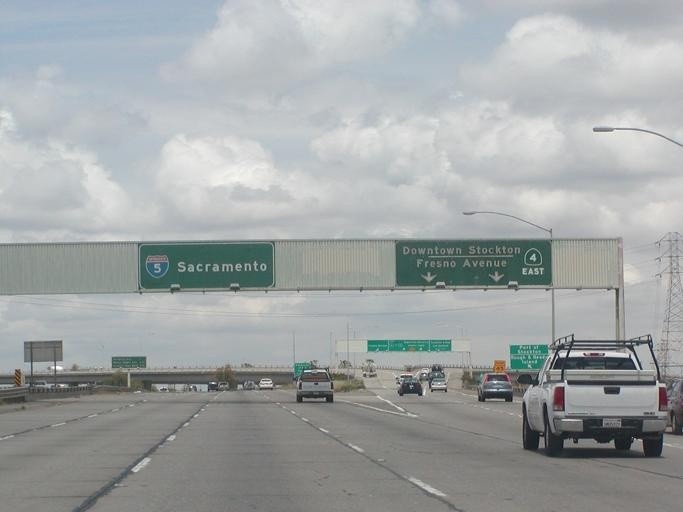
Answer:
left=666, top=378, right=683, bottom=436
left=430, top=378, right=447, bottom=392
left=395, top=367, right=429, bottom=396
left=243, top=377, right=273, bottom=390
left=207, top=381, right=229, bottom=392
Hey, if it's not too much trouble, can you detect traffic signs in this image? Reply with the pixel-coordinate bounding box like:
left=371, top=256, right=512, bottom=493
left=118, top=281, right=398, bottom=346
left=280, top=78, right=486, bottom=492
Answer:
left=508, top=344, right=548, bottom=371
left=365, top=339, right=451, bottom=353
left=394, top=238, right=550, bottom=289
left=137, top=239, right=274, bottom=291
left=111, top=356, right=146, bottom=369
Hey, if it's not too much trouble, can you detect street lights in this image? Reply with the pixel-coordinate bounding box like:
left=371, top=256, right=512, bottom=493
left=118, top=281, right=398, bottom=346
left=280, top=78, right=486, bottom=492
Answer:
left=460, top=209, right=555, bottom=342
left=590, top=124, right=680, bottom=150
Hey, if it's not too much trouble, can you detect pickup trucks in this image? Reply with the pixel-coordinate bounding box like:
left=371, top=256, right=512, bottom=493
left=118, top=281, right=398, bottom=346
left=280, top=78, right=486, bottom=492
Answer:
left=515, top=335, right=669, bottom=457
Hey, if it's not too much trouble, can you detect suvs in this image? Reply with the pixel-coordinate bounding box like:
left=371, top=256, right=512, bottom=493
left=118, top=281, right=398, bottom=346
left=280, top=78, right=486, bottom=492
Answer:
left=294, top=368, right=333, bottom=402
left=475, top=373, right=513, bottom=402
left=32, top=380, right=47, bottom=387
